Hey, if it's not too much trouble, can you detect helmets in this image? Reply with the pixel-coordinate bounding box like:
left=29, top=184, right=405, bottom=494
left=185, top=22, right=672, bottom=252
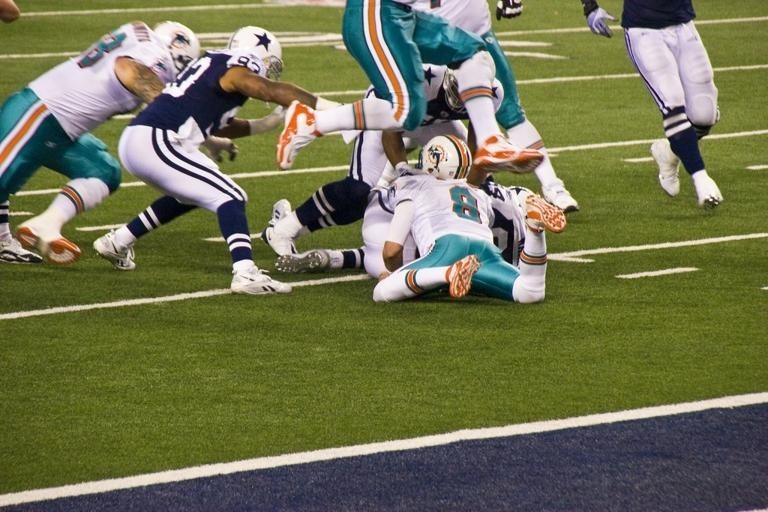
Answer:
left=417, top=133, right=472, bottom=181
left=152, top=18, right=200, bottom=76
left=442, top=71, right=505, bottom=119
left=228, top=24, right=284, bottom=80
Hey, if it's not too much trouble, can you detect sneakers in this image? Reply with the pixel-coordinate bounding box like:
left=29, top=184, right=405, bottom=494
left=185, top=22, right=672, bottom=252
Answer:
left=276, top=99, right=317, bottom=171
left=693, top=175, right=725, bottom=211
left=228, top=266, right=293, bottom=296
left=524, top=193, right=567, bottom=234
left=262, top=197, right=300, bottom=254
left=93, top=228, right=137, bottom=273
left=12, top=222, right=82, bottom=266
left=1, top=237, right=44, bottom=265
left=472, top=132, right=544, bottom=175
left=448, top=252, right=480, bottom=299
left=650, top=137, right=683, bottom=197
left=275, top=247, right=329, bottom=273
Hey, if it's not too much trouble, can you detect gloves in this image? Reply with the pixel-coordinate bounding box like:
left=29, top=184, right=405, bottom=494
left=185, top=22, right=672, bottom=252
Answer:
left=495, top=0, right=524, bottom=19
left=582, top=5, right=618, bottom=38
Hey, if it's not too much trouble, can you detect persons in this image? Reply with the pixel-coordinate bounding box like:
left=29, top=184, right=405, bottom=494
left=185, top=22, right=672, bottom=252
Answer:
left=1, top=21, right=351, bottom=295
left=581, top=1, right=724, bottom=210
left=261, top=1, right=579, bottom=303
